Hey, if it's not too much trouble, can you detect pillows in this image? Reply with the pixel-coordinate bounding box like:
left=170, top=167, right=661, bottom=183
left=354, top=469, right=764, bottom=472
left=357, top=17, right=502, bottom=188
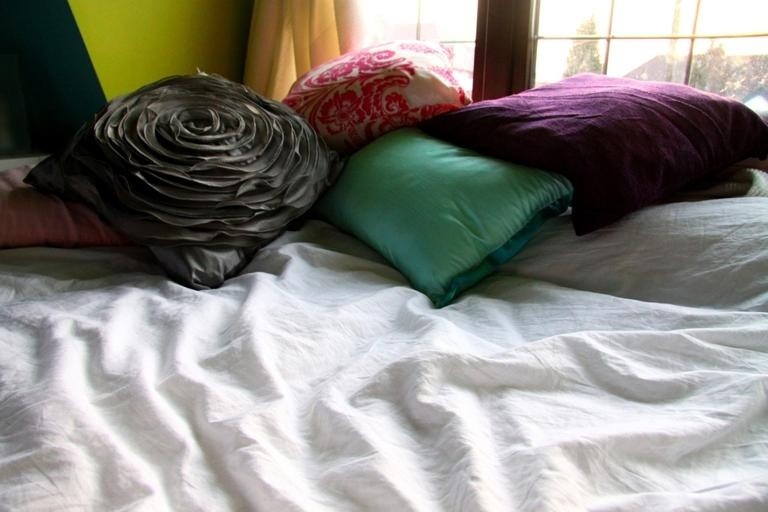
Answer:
left=280, top=42, right=469, bottom=155
left=313, top=127, right=574, bottom=306
left=25, top=69, right=335, bottom=289
left=422, top=72, right=766, bottom=235
